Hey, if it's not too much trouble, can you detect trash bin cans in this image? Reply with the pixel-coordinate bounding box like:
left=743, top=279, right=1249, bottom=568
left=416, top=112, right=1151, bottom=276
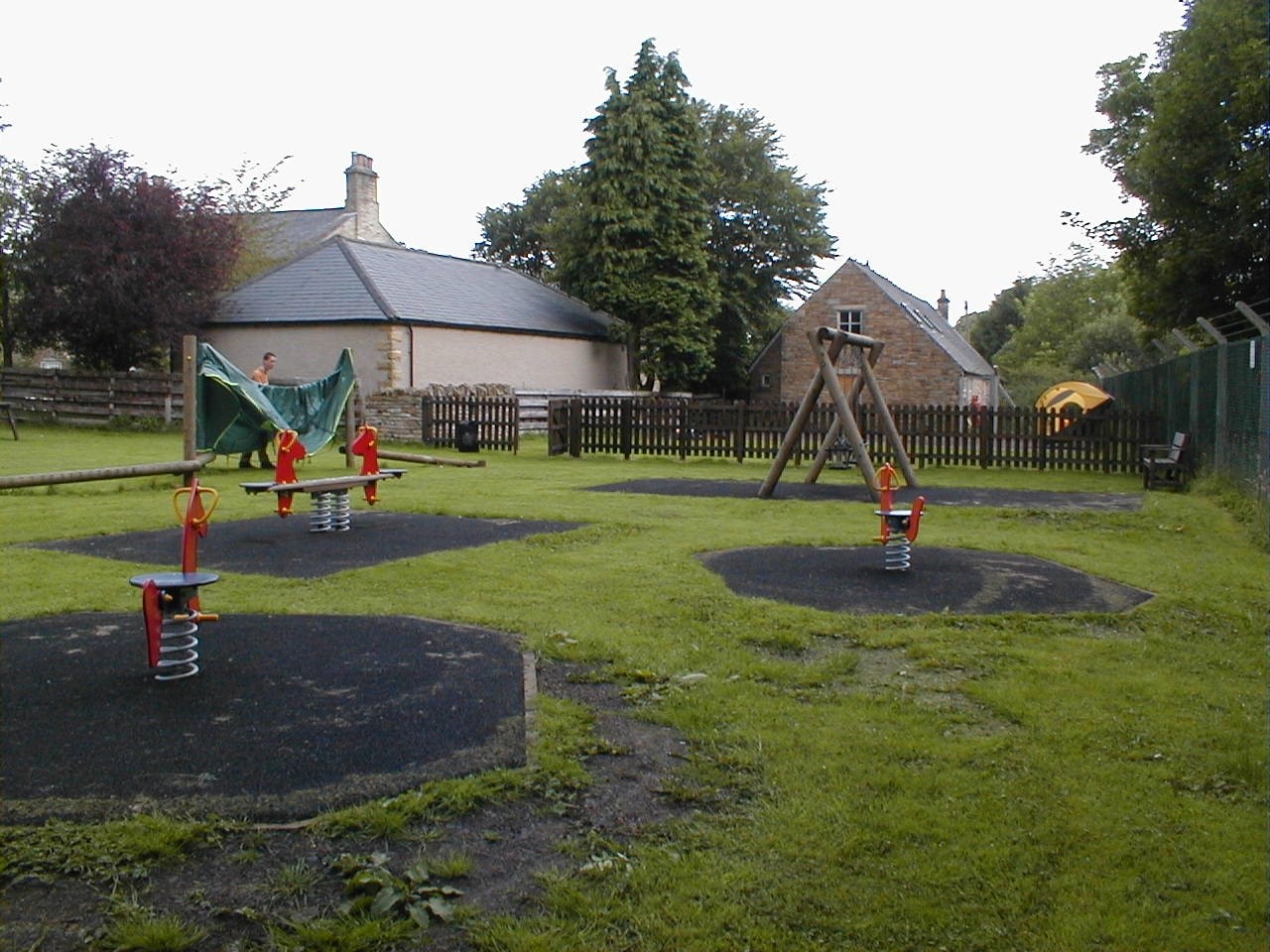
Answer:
left=456, top=421, right=479, bottom=452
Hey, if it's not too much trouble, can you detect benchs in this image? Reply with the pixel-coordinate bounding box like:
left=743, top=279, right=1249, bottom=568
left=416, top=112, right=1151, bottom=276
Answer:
left=1138, top=428, right=1192, bottom=494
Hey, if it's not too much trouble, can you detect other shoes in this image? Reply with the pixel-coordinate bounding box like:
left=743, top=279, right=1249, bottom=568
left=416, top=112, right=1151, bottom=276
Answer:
left=240, top=461, right=254, bottom=468
left=261, top=461, right=275, bottom=468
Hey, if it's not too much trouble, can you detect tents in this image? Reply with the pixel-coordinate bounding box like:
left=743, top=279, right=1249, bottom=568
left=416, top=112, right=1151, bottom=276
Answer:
left=1032, top=380, right=1115, bottom=444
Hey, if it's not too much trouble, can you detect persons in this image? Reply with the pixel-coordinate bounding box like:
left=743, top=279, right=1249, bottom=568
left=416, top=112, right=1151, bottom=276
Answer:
left=235, top=352, right=277, bottom=470
